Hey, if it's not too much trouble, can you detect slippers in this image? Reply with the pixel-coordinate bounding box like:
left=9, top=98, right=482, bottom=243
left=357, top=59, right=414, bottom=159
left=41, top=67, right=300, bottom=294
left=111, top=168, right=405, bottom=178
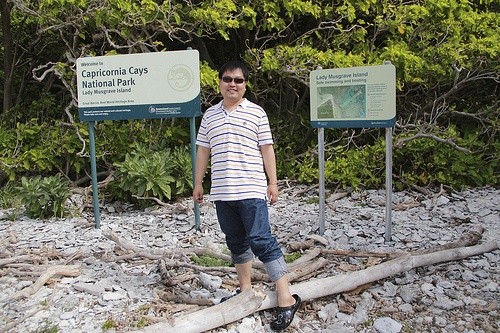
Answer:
left=270, top=294, right=301, bottom=330
left=220, top=289, right=241, bottom=303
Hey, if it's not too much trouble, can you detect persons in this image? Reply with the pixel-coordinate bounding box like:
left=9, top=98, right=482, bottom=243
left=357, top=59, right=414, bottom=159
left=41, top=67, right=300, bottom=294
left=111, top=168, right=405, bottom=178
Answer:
left=193, top=60, right=302, bottom=331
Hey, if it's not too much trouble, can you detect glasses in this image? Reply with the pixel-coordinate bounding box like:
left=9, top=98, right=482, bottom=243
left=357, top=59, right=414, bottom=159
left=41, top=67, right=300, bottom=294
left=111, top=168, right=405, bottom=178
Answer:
left=222, top=76, right=247, bottom=83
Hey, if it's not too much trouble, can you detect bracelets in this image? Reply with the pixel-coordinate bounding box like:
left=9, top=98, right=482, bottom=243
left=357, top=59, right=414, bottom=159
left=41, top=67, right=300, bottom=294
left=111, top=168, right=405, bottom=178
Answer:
left=268, top=182, right=277, bottom=186
